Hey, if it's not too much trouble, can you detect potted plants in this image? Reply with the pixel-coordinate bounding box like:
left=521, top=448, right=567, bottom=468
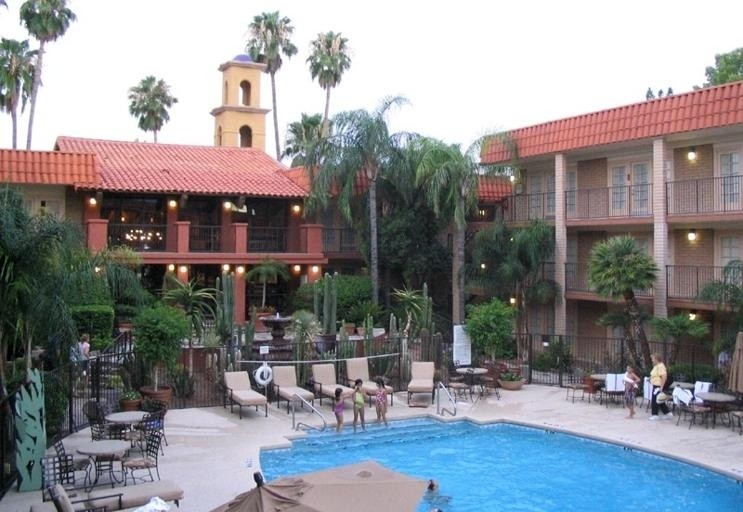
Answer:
left=460, top=293, right=522, bottom=389
left=495, top=370, right=528, bottom=391
left=128, top=300, right=193, bottom=405
left=117, top=387, right=143, bottom=412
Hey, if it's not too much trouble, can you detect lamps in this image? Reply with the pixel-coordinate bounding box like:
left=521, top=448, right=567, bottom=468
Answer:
left=686, top=146, right=696, bottom=161
left=688, top=309, right=696, bottom=321
left=687, top=228, right=696, bottom=241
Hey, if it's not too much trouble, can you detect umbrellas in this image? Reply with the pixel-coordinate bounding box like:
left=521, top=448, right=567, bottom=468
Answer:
left=728, top=332, right=742, bottom=396
left=211, top=460, right=438, bottom=512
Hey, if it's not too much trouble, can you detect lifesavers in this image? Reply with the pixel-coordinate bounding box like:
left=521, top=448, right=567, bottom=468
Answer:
left=256, top=366, right=273, bottom=385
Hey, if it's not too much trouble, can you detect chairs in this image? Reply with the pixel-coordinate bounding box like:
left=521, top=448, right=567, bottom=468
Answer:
left=560, top=367, right=742, bottom=437
left=219, top=356, right=502, bottom=421
left=28, top=392, right=184, bottom=512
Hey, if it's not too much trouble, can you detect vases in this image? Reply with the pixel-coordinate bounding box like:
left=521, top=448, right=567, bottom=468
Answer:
left=342, top=324, right=355, bottom=336
left=355, top=327, right=366, bottom=337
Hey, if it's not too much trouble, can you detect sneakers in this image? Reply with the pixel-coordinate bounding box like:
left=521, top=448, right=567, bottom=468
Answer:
left=648, top=415, right=661, bottom=420
left=661, top=411, right=673, bottom=420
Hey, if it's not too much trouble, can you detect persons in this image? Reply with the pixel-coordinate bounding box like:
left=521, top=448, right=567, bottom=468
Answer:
left=351, top=379, right=368, bottom=433
left=335, top=388, right=352, bottom=433
left=79, top=333, right=92, bottom=372
left=374, top=378, right=388, bottom=427
left=648, top=352, right=673, bottom=420
left=621, top=364, right=641, bottom=419
